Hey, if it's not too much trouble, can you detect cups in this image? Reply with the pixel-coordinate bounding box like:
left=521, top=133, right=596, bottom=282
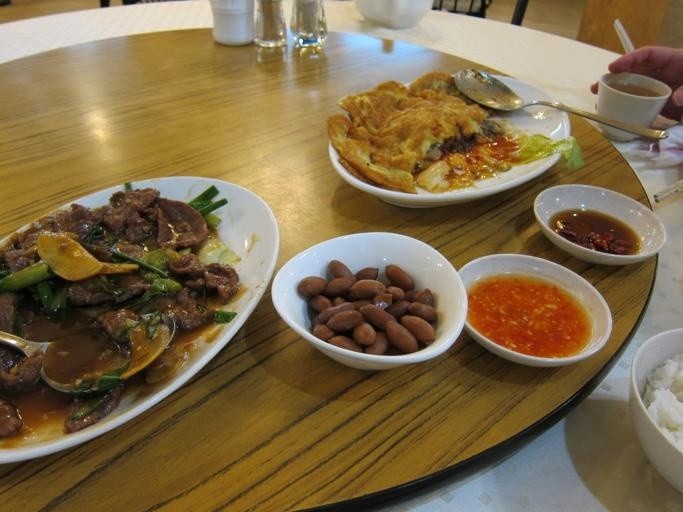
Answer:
left=209, top=0, right=255, bottom=46
left=594, top=70, right=673, bottom=141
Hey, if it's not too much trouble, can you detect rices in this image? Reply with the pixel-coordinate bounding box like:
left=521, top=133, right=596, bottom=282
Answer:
left=643, top=354, right=683, bottom=450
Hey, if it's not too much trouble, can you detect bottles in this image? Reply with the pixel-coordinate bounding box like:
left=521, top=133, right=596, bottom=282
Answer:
left=256, top=0, right=329, bottom=50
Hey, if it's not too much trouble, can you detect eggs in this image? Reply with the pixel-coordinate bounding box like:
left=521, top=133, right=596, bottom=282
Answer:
left=326, top=71, right=492, bottom=193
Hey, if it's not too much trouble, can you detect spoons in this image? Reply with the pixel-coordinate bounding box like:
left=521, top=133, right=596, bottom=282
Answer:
left=454, top=68, right=669, bottom=144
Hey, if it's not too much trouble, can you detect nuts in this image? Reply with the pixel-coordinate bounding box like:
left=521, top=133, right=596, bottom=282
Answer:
left=299, top=260, right=437, bottom=355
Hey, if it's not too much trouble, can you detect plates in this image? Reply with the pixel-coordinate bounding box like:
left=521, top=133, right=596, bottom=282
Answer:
left=326, top=72, right=571, bottom=210
left=275, top=234, right=469, bottom=370
left=2, top=177, right=279, bottom=461
left=458, top=181, right=669, bottom=366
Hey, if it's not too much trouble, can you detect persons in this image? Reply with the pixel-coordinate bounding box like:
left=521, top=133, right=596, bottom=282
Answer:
left=590, top=45, right=683, bottom=121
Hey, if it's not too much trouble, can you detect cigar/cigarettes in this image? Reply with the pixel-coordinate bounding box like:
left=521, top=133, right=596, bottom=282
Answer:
left=614, top=19, right=634, bottom=54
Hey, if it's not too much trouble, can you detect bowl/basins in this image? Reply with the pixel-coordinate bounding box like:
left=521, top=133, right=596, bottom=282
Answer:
left=631, top=329, right=682, bottom=490
